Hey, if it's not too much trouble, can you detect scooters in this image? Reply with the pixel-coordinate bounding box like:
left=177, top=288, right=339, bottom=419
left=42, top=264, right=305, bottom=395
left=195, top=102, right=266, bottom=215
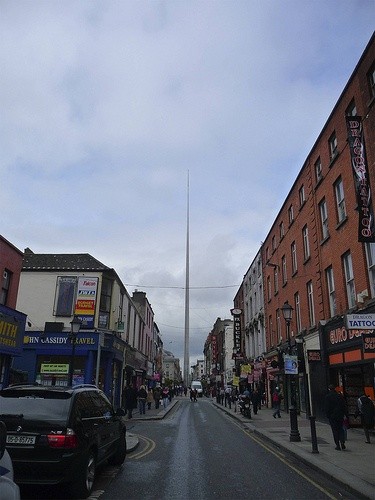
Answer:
left=237, top=394, right=252, bottom=420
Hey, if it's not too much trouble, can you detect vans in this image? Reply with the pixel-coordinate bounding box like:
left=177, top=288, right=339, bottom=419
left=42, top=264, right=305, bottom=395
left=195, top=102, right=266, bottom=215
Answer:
left=191, top=381, right=203, bottom=397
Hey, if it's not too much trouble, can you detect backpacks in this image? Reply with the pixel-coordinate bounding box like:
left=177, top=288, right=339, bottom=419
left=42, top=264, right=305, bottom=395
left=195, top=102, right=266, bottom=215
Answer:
left=273, top=392, right=279, bottom=402
left=360, top=396, right=375, bottom=415
left=138, top=388, right=147, bottom=398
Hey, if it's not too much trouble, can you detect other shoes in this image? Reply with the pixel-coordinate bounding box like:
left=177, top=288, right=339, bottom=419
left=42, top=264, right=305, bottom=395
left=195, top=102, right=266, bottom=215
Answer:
left=335, top=446, right=340, bottom=450
left=365, top=441, right=370, bottom=443
left=342, top=445, right=345, bottom=449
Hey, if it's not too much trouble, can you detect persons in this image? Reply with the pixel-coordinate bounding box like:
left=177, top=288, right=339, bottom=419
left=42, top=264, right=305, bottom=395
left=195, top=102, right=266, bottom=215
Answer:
left=190, top=387, right=198, bottom=403
left=146, top=388, right=153, bottom=410
left=272, top=387, right=281, bottom=418
left=217, top=385, right=262, bottom=416
left=335, top=386, right=348, bottom=441
left=357, top=391, right=375, bottom=444
left=165, top=383, right=188, bottom=398
left=154, top=386, right=169, bottom=408
left=138, top=385, right=148, bottom=415
left=324, top=384, right=347, bottom=450
left=122, top=384, right=138, bottom=418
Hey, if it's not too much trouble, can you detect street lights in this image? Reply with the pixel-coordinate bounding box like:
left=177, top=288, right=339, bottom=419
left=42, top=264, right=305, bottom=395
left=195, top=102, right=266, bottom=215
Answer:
left=94, top=330, right=125, bottom=387
left=280, top=300, right=301, bottom=442
left=67, top=316, right=83, bottom=386
left=232, top=346, right=239, bottom=395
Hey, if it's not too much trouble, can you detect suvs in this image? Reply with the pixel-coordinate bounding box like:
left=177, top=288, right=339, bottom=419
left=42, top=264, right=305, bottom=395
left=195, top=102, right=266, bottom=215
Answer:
left=0, top=382, right=128, bottom=500
